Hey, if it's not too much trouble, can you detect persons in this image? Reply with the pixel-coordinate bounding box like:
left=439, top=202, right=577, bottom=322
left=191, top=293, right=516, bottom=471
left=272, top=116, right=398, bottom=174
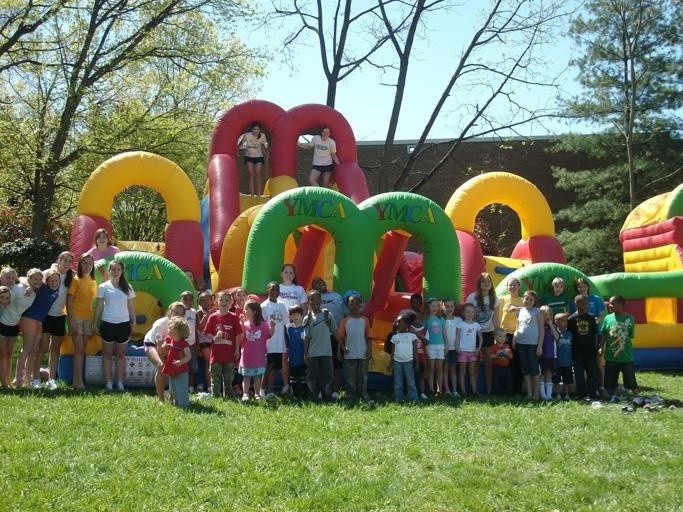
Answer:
left=196, top=291, right=220, bottom=362
left=93, top=260, right=136, bottom=391
left=145, top=302, right=187, bottom=406
left=569, top=275, right=605, bottom=322
left=488, top=326, right=514, bottom=400
left=454, top=303, right=484, bottom=396
left=304, top=289, right=350, bottom=402
left=298, top=128, right=339, bottom=189
left=260, top=280, right=291, bottom=396
left=155, top=316, right=191, bottom=408
left=498, top=277, right=525, bottom=344
left=409, top=311, right=430, bottom=400
left=236, top=125, right=270, bottom=198
left=204, top=290, right=243, bottom=403
left=65, top=253, right=97, bottom=391
left=240, top=300, right=278, bottom=402
left=233, top=288, right=248, bottom=315
left=544, top=277, right=571, bottom=319
left=184, top=268, right=194, bottom=284
left=0, top=268, right=43, bottom=390
left=334, top=295, right=375, bottom=403
left=0, top=267, right=18, bottom=289
left=443, top=299, right=460, bottom=397
left=13, top=270, right=61, bottom=388
left=425, top=298, right=448, bottom=396
left=0, top=284, right=11, bottom=309
left=286, top=304, right=310, bottom=400
left=568, top=295, right=604, bottom=403
left=600, top=295, right=641, bottom=403
left=552, top=312, right=573, bottom=400
left=391, top=294, right=422, bottom=334
left=512, top=290, right=545, bottom=401
left=537, top=306, right=557, bottom=401
left=31, top=251, right=74, bottom=388
left=181, top=291, right=197, bottom=393
left=277, top=263, right=306, bottom=308
left=309, top=277, right=351, bottom=330
left=86, top=228, right=120, bottom=260
left=391, top=314, right=421, bottom=405
left=465, top=272, right=501, bottom=353
left=250, top=293, right=276, bottom=400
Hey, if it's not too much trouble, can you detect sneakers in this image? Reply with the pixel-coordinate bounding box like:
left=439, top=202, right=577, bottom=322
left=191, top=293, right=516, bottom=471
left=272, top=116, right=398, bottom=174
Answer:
left=32, top=380, right=41, bottom=389
left=542, top=394, right=547, bottom=401
left=281, top=385, right=290, bottom=395
left=452, top=391, right=461, bottom=397
left=565, top=395, right=569, bottom=400
left=446, top=391, right=452, bottom=396
left=557, top=395, right=561, bottom=400
left=198, top=385, right=203, bottom=395
left=421, top=393, right=427, bottom=401
left=190, top=387, right=194, bottom=393
left=46, top=382, right=57, bottom=390
left=242, top=393, right=249, bottom=401
left=255, top=394, right=260, bottom=400
left=105, top=381, right=113, bottom=391
left=118, top=382, right=124, bottom=392
left=547, top=395, right=552, bottom=401
